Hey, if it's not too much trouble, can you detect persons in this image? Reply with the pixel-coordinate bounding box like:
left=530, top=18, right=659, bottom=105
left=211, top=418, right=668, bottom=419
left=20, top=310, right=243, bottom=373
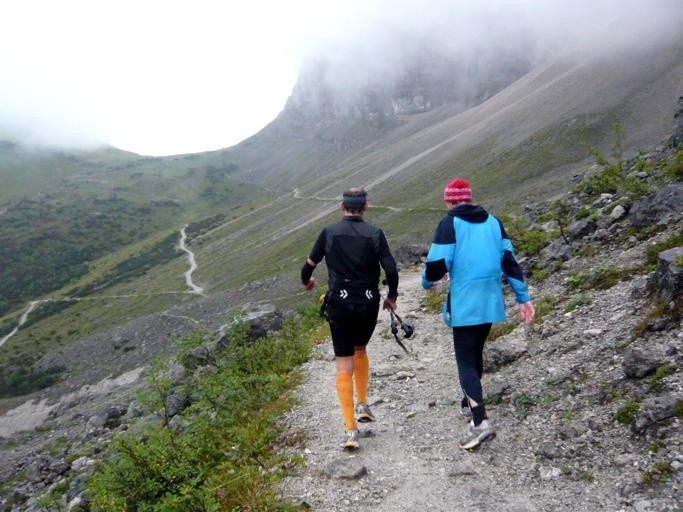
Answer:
left=418, top=177, right=536, bottom=451
left=300, top=186, right=399, bottom=451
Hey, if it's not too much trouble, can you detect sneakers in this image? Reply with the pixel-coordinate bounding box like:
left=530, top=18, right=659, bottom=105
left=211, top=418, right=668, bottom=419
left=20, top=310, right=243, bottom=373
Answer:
left=458, top=420, right=495, bottom=450
left=460, top=405, right=472, bottom=422
left=355, top=403, right=375, bottom=422
left=339, top=430, right=359, bottom=449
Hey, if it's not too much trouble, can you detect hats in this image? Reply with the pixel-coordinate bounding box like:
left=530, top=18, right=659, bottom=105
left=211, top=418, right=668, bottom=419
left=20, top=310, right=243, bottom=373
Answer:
left=444, top=180, right=471, bottom=202
